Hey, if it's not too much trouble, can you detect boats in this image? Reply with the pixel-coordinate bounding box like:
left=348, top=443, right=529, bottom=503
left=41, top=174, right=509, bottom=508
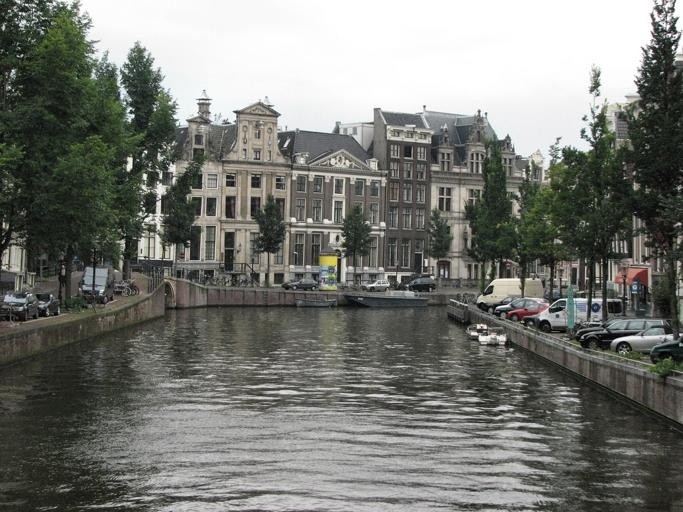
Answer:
left=465, top=323, right=507, bottom=346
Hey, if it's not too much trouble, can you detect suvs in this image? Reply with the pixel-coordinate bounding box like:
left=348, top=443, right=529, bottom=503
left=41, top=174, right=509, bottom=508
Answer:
left=0, top=292, right=41, bottom=322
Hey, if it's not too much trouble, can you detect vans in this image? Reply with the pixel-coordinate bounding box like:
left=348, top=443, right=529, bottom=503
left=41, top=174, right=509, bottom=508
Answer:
left=77, top=263, right=116, bottom=305
left=536, top=297, right=625, bottom=335
left=475, top=276, right=544, bottom=312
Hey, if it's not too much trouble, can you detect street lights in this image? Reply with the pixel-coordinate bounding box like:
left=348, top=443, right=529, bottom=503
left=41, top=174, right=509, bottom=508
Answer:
left=557, top=266, right=565, bottom=297
left=618, top=261, right=628, bottom=318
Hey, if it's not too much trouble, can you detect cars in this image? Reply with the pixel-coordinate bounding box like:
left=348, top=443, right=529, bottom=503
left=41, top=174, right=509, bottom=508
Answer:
left=33, top=292, right=65, bottom=316
left=573, top=315, right=683, bottom=365
left=282, top=277, right=318, bottom=290
left=399, top=276, right=436, bottom=293
left=362, top=279, right=391, bottom=292
left=493, top=293, right=552, bottom=322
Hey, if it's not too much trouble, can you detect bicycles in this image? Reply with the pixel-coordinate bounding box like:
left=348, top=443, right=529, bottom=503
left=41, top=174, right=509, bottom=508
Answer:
left=114, top=279, right=140, bottom=297
